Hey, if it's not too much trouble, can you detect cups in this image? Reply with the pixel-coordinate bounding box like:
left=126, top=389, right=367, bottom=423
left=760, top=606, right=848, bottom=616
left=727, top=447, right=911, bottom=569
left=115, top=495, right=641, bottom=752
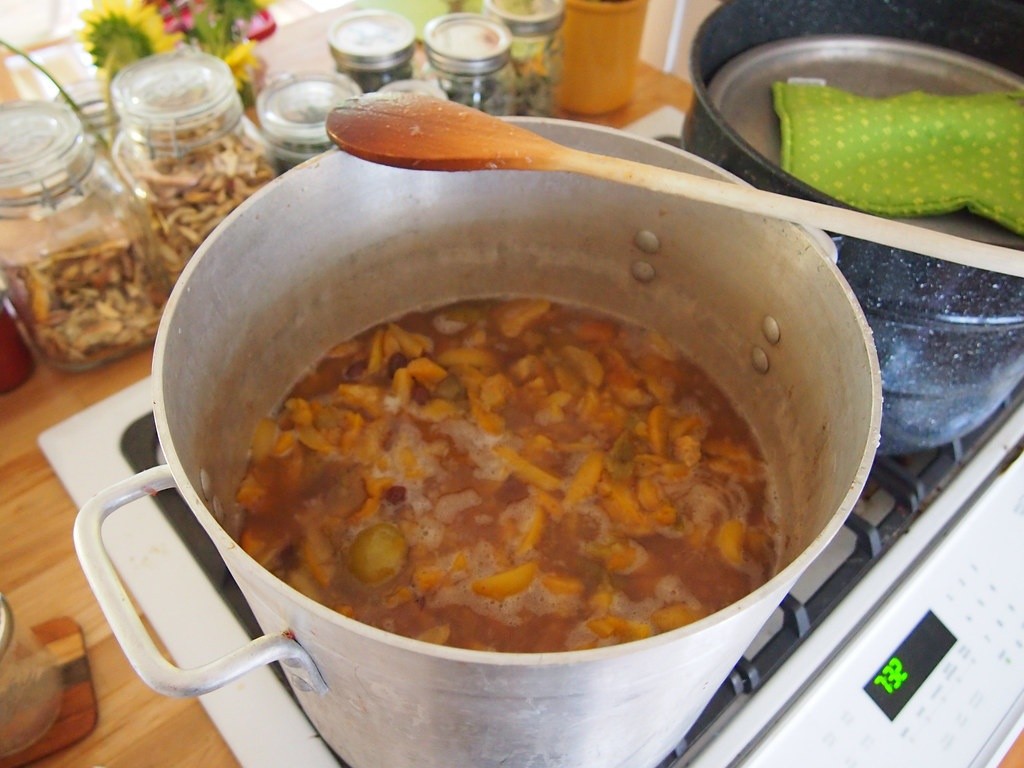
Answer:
left=1, top=592, right=62, bottom=756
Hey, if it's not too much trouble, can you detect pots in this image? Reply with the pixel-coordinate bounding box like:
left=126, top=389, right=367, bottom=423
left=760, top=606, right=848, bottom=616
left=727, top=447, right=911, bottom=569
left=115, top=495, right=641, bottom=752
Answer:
left=72, top=117, right=883, bottom=768
left=679, top=0, right=1023, bottom=459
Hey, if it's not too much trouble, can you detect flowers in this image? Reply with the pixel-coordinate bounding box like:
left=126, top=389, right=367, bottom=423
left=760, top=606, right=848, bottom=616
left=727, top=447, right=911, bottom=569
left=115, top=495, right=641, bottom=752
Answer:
left=74, top=0, right=276, bottom=148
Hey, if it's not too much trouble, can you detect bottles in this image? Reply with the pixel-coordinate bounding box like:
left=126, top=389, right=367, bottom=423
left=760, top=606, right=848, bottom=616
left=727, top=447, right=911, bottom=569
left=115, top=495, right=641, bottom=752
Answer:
left=256, top=67, right=361, bottom=178
left=476, top=0, right=574, bottom=116
left=0, top=104, right=172, bottom=374
left=112, top=42, right=273, bottom=294
left=323, top=9, right=416, bottom=105
left=421, top=11, right=516, bottom=120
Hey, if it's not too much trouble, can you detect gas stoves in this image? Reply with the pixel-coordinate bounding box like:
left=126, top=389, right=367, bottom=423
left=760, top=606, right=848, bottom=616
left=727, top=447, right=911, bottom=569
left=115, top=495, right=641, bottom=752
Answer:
left=30, top=97, right=1024, bottom=768
